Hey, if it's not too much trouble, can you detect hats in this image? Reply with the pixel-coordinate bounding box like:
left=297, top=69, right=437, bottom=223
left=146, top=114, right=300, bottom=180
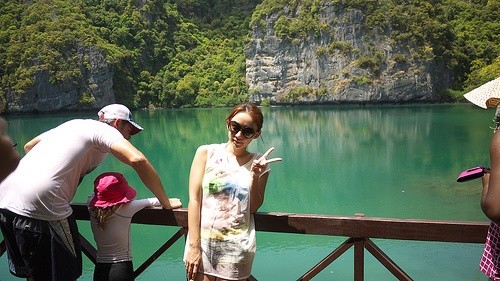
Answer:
left=89, top=171, right=137, bottom=208
left=463, top=76, right=500, bottom=109
left=98, top=104, right=144, bottom=136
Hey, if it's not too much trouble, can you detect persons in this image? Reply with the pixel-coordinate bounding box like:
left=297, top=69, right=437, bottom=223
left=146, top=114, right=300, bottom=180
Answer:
left=183, top=103, right=282, bottom=281
left=0, top=104, right=182, bottom=281
left=463, top=77, right=500, bottom=281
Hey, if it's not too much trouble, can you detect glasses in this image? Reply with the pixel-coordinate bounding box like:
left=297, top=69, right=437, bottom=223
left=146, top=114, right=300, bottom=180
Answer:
left=227, top=120, right=258, bottom=139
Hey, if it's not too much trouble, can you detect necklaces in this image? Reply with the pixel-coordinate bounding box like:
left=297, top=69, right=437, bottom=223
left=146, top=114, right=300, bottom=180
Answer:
left=247, top=153, right=252, bottom=157
